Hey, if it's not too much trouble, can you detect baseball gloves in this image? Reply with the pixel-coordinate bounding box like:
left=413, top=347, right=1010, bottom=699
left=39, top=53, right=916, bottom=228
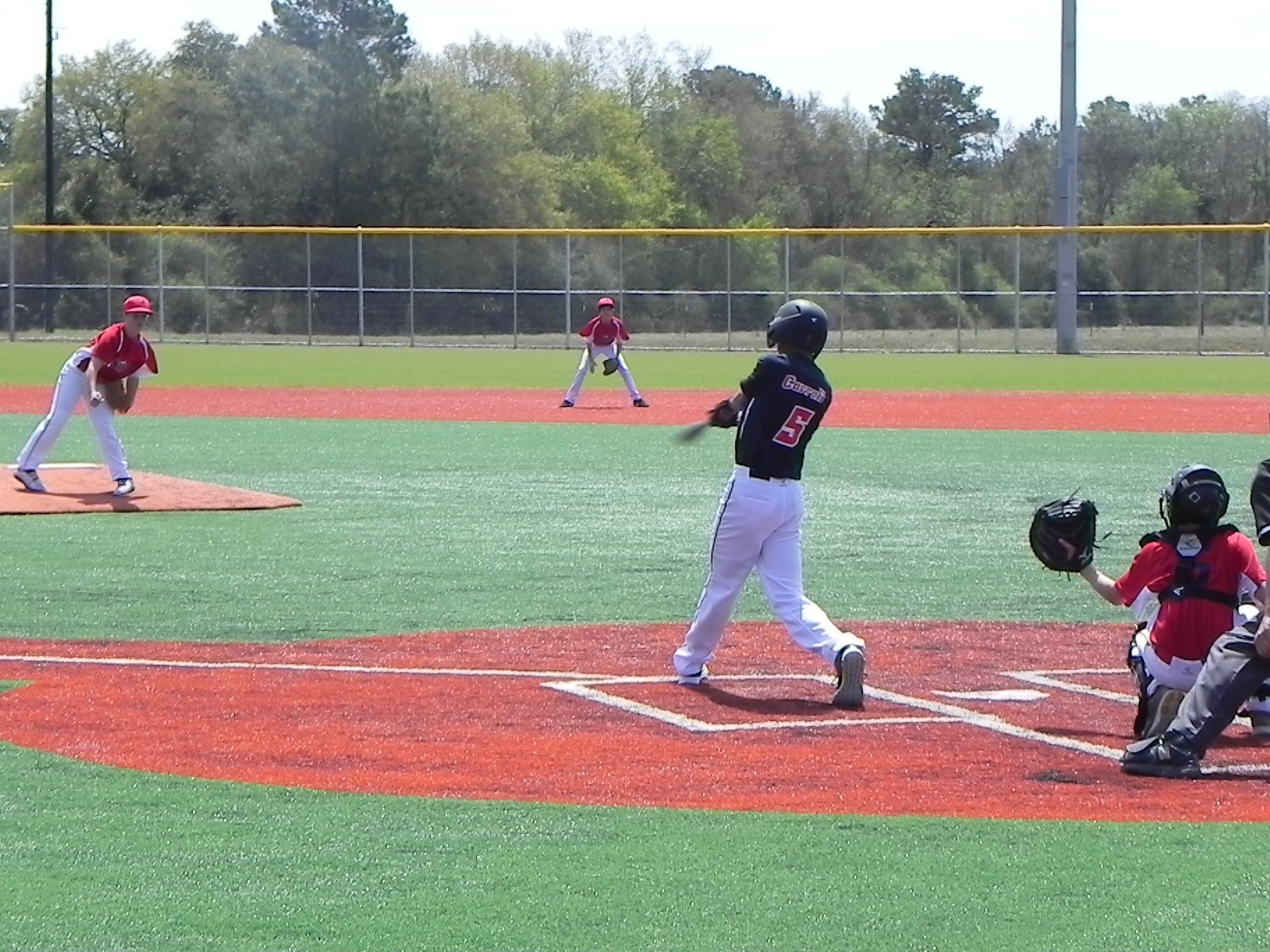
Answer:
left=1028, top=499, right=1099, bottom=572
left=603, top=359, right=619, bottom=376
left=105, top=377, right=130, bottom=414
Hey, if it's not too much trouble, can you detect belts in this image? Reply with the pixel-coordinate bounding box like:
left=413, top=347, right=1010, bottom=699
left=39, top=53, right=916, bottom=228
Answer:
left=749, top=469, right=770, bottom=481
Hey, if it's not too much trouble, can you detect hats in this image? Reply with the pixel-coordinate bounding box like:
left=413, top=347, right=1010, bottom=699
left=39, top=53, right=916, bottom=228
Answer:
left=123, top=296, right=153, bottom=315
left=597, top=298, right=615, bottom=309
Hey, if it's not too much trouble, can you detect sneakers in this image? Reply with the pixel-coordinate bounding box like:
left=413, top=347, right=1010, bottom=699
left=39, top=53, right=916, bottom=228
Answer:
left=832, top=645, right=863, bottom=706
left=1250, top=713, right=1270, bottom=735
left=679, top=663, right=707, bottom=684
left=113, top=479, right=134, bottom=496
left=14, top=468, right=45, bottom=492
left=1141, top=686, right=1183, bottom=739
left=1122, top=735, right=1201, bottom=777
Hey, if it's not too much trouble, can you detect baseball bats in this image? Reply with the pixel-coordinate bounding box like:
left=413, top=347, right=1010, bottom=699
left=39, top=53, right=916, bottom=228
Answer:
left=674, top=419, right=712, bottom=449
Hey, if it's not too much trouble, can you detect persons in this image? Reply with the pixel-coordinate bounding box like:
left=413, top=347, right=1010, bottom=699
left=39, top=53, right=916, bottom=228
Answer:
left=673, top=298, right=866, bottom=709
left=1059, top=459, right=1270, bottom=747
left=1122, top=460, right=1270, bottom=778
left=559, top=297, right=650, bottom=407
left=13, top=296, right=158, bottom=500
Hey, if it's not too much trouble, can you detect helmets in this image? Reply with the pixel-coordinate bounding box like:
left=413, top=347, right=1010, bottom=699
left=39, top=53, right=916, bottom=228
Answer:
left=768, top=299, right=827, bottom=353
left=1172, top=472, right=1220, bottom=521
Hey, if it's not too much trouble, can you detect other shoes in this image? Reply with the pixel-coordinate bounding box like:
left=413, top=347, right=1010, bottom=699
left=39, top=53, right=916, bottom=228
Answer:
left=633, top=399, right=649, bottom=407
left=560, top=400, right=574, bottom=407
